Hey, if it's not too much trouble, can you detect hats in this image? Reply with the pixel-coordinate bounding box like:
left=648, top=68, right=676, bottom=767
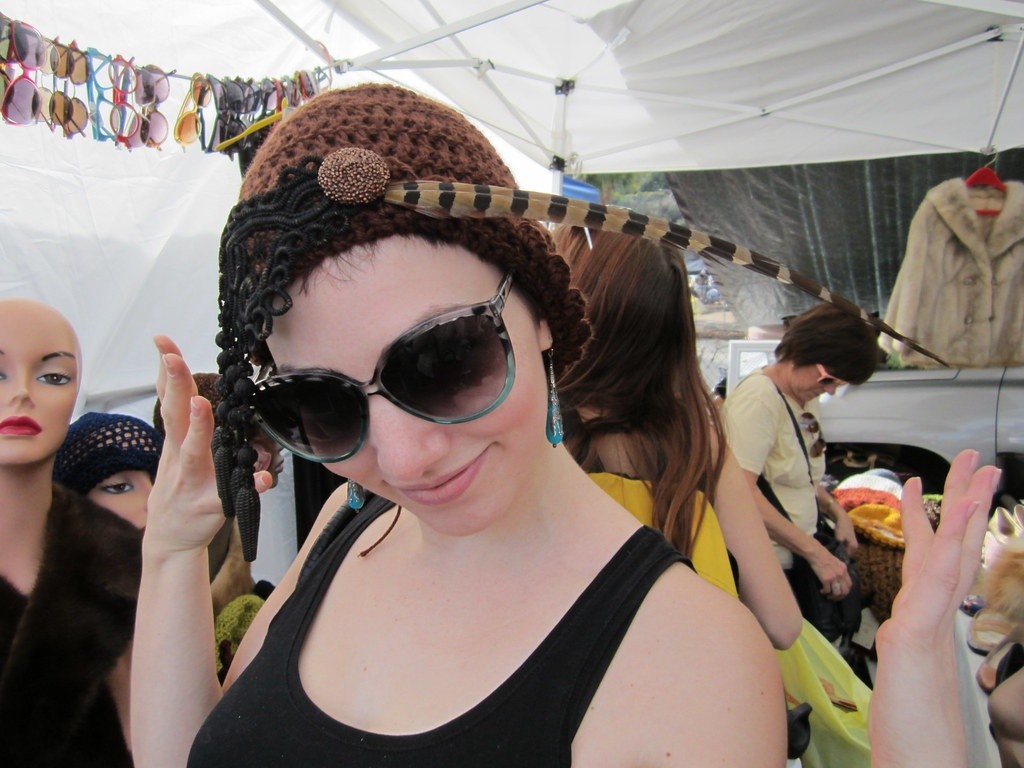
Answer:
left=52, top=411, right=163, bottom=498
left=152, top=373, right=280, bottom=463
left=239, top=83, right=592, bottom=376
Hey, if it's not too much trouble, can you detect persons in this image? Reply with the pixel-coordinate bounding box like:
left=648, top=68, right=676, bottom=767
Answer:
left=0, top=298, right=138, bottom=768
left=53, top=413, right=159, bottom=533
left=154, top=373, right=284, bottom=585
left=130, top=83, right=1004, bottom=768
left=988, top=666, right=1024, bottom=768
left=550, top=220, right=803, bottom=651
left=724, top=303, right=879, bottom=642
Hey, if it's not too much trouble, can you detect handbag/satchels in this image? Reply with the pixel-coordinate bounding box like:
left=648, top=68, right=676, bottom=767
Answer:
left=791, top=517, right=862, bottom=639
left=771, top=615, right=875, bottom=768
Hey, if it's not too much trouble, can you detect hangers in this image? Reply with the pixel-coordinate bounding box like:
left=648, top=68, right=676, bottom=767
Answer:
left=964, top=147, right=1008, bottom=215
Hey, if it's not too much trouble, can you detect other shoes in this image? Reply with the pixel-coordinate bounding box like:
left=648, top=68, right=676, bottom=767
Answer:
left=959, top=507, right=1024, bottom=737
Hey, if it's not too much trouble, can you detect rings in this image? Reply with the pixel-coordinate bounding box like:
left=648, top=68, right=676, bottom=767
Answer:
left=831, top=583, right=841, bottom=596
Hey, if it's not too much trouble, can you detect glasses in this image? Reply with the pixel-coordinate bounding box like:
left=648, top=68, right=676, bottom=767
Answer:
left=799, top=411, right=828, bottom=459
left=816, top=363, right=848, bottom=387
left=1, top=12, right=326, bottom=158
left=251, top=274, right=517, bottom=463
